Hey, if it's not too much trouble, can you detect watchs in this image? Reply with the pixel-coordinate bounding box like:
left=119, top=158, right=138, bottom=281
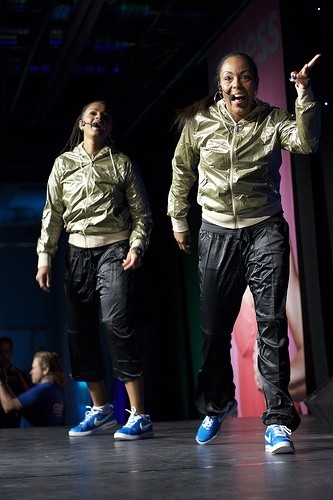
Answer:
left=134, top=247, right=143, bottom=256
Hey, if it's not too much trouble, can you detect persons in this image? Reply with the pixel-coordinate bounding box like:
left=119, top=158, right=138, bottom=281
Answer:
left=0, top=351, right=67, bottom=427
left=36, top=100, right=154, bottom=441
left=0, top=337, right=31, bottom=428
left=167, top=53, right=321, bottom=455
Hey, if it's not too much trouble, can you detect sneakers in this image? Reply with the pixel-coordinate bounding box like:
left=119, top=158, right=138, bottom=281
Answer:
left=114, top=406, right=157, bottom=441
left=68, top=403, right=118, bottom=437
left=263, top=424, right=295, bottom=454
left=195, top=397, right=238, bottom=444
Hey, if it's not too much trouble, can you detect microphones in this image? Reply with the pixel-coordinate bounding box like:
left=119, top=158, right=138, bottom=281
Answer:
left=218, top=85, right=236, bottom=101
left=82, top=122, right=98, bottom=127
